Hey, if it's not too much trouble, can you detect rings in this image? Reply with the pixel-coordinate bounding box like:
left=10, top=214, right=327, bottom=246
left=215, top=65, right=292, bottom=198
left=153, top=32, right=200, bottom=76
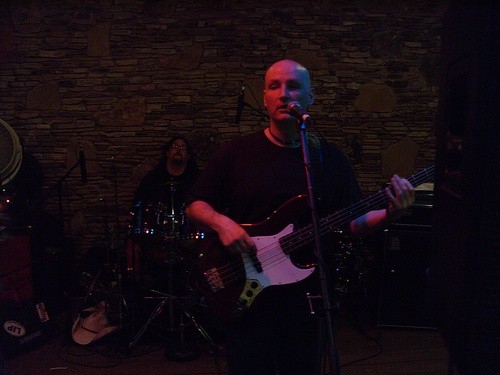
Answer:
left=399, top=193, right=404, bottom=202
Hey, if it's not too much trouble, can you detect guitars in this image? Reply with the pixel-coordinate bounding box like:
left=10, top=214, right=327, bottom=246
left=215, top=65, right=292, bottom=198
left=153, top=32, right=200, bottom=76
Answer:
left=193, top=165, right=437, bottom=328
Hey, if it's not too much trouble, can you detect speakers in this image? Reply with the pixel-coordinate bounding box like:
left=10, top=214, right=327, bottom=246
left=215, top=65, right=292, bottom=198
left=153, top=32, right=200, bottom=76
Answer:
left=377, top=227, right=441, bottom=330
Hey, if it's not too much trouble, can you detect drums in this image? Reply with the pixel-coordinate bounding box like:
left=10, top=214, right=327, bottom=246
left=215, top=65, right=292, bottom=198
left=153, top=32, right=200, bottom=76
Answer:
left=175, top=205, right=208, bottom=246
left=335, top=229, right=365, bottom=290
left=130, top=201, right=171, bottom=243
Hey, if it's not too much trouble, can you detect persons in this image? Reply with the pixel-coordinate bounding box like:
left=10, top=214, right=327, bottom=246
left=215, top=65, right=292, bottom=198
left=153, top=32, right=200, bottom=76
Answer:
left=427, top=128, right=469, bottom=175
left=179, top=60, right=413, bottom=375
left=137, top=138, right=197, bottom=258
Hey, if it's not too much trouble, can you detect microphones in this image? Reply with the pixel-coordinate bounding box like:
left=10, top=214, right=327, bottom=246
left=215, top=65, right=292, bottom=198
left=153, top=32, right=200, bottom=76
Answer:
left=79, top=150, right=88, bottom=183
left=235, top=87, right=245, bottom=124
left=287, top=101, right=310, bottom=124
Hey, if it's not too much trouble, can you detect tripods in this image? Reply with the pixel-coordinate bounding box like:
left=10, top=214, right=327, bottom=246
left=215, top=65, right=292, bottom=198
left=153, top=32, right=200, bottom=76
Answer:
left=69, top=177, right=223, bottom=353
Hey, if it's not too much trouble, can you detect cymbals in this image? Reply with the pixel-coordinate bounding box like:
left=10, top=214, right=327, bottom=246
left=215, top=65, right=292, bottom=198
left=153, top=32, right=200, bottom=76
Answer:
left=80, top=206, right=130, bottom=217
left=87, top=219, right=127, bottom=223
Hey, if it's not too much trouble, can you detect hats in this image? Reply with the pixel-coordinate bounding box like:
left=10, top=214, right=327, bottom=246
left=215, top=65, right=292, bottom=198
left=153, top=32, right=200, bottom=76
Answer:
left=70, top=311, right=118, bottom=345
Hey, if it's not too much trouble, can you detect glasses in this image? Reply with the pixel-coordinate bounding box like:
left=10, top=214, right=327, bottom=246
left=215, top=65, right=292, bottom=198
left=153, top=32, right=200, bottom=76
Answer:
left=170, top=143, right=187, bottom=150
